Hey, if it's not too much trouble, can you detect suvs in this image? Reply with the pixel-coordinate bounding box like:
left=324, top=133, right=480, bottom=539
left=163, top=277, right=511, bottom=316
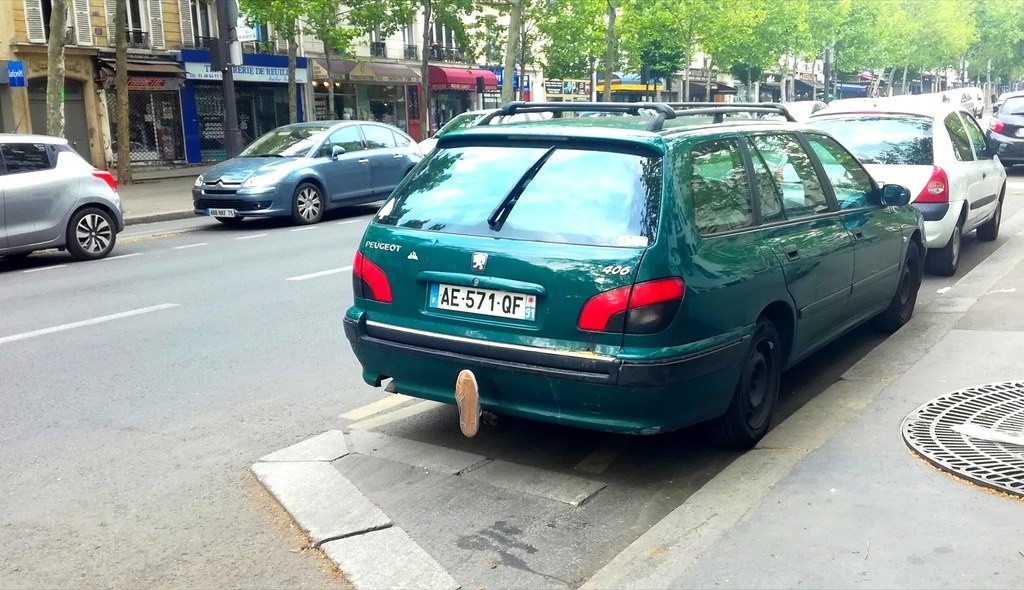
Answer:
left=987, top=91, right=1024, bottom=174
left=343, top=101, right=925, bottom=449
left=801, top=108, right=1008, bottom=278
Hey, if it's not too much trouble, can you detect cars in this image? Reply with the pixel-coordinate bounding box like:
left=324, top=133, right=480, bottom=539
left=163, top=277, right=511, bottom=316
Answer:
left=192, top=121, right=423, bottom=225
left=923, top=87, right=984, bottom=118
left=767, top=101, right=826, bottom=123
left=416, top=107, right=547, bottom=160
left=0, top=132, right=124, bottom=261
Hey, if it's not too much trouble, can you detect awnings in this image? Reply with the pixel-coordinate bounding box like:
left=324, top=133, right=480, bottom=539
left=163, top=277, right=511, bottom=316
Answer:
left=105, top=62, right=189, bottom=73
left=597, top=72, right=662, bottom=91
left=689, top=82, right=737, bottom=96
left=313, top=59, right=421, bottom=83
left=428, top=67, right=497, bottom=90
left=795, top=80, right=834, bottom=93
left=761, top=83, right=787, bottom=91
left=839, top=85, right=872, bottom=93
